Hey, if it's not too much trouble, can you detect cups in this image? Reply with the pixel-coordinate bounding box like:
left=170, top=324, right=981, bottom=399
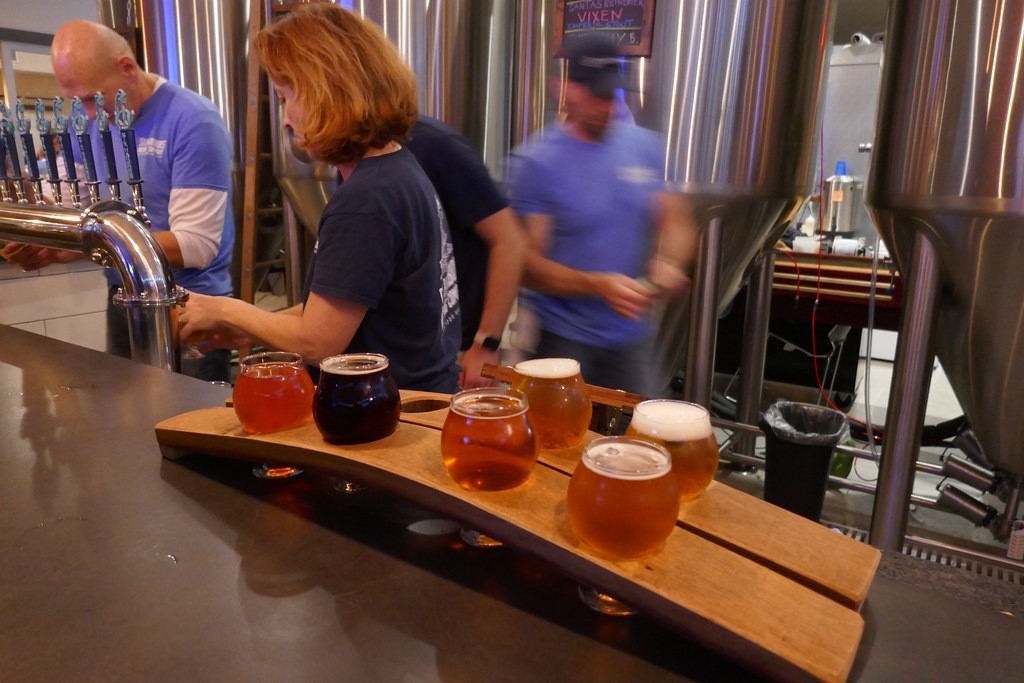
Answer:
left=180, top=327, right=212, bottom=359
left=440, top=387, right=541, bottom=491
left=623, top=399, right=720, bottom=502
left=231, top=350, right=315, bottom=434
left=312, top=352, right=402, bottom=445
left=509, top=357, right=593, bottom=452
left=565, top=436, right=681, bottom=560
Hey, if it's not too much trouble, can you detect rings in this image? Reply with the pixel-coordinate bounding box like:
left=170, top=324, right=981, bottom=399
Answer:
left=179, top=317, right=182, bottom=324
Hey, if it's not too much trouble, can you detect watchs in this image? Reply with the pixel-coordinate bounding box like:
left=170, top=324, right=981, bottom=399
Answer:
left=473, top=333, right=503, bottom=351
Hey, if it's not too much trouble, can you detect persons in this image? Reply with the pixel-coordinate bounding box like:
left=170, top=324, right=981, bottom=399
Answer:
left=175, top=3, right=466, bottom=394
left=0, top=19, right=234, bottom=383
left=338, top=114, right=526, bottom=390
left=506, top=31, right=696, bottom=393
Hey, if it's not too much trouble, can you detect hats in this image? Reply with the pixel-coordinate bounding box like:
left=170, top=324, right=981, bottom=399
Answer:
left=565, top=31, right=624, bottom=94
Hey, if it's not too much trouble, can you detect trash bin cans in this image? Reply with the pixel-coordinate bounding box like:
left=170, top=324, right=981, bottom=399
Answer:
left=757, top=401, right=851, bottom=524
